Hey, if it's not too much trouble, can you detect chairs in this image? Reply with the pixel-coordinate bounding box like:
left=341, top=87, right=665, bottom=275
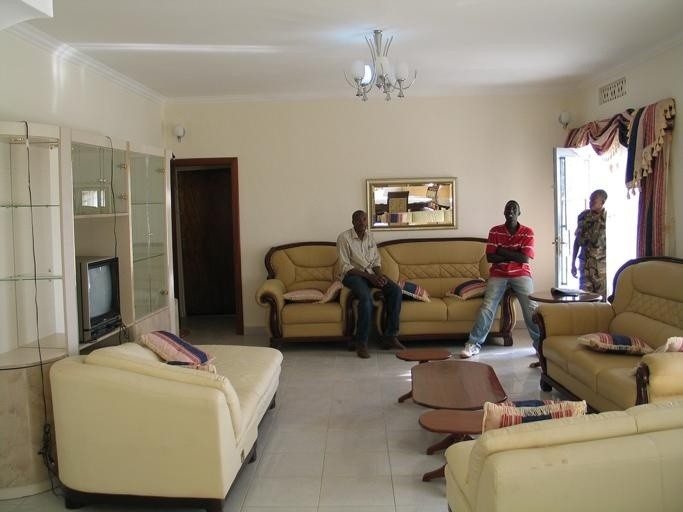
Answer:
left=424, top=184, right=440, bottom=210
left=385, top=187, right=409, bottom=212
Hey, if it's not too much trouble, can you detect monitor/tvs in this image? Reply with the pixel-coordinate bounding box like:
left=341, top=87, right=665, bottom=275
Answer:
left=75, top=255, right=122, bottom=343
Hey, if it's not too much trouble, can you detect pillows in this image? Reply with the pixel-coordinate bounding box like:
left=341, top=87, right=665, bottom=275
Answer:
left=312, top=277, right=343, bottom=304
left=624, top=334, right=683, bottom=379
left=412, top=209, right=444, bottom=224
left=394, top=278, right=433, bottom=304
left=381, top=212, right=412, bottom=226
left=442, top=276, right=487, bottom=300
left=280, top=282, right=325, bottom=304
left=575, top=330, right=654, bottom=356
left=138, top=328, right=218, bottom=372
left=479, top=397, right=587, bottom=436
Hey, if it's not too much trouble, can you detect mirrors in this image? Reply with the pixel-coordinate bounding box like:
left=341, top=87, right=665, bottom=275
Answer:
left=363, top=177, right=458, bottom=232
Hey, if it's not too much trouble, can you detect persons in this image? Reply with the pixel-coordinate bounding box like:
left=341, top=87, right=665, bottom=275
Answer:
left=460, top=200, right=541, bottom=357
left=336, top=210, right=405, bottom=359
left=571, top=189, right=608, bottom=302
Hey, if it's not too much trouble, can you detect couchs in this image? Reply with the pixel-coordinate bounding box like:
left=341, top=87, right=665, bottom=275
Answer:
left=443, top=397, right=683, bottom=512
left=253, top=240, right=358, bottom=352
left=529, top=255, right=683, bottom=414
left=369, top=236, right=516, bottom=346
left=47, top=342, right=284, bottom=511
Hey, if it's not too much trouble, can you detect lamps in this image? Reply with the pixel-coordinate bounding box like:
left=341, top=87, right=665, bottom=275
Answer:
left=339, top=29, right=419, bottom=104
left=173, top=121, right=187, bottom=143
left=556, top=108, right=574, bottom=132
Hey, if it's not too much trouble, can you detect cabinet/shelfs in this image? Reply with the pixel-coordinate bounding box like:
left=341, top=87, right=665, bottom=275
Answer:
left=0, top=119, right=179, bottom=502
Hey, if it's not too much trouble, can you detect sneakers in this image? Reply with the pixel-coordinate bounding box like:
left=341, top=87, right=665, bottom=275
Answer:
left=461, top=343, right=479, bottom=357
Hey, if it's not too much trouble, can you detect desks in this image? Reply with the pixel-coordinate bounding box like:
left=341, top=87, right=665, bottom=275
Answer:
left=416, top=409, right=485, bottom=483
left=525, top=289, right=603, bottom=369
left=408, top=360, right=507, bottom=410
left=393, top=348, right=453, bottom=403
